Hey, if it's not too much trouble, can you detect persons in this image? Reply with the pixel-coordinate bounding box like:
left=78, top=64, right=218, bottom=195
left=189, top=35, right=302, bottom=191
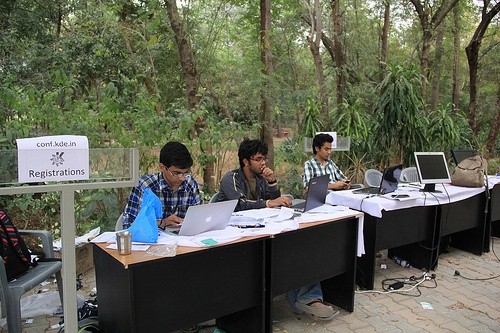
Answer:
left=302, top=134, right=388, bottom=273
left=122, top=141, right=200, bottom=333
left=214, top=137, right=339, bottom=324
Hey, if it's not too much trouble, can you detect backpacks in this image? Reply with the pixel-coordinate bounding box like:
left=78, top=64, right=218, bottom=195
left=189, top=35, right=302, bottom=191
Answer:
left=0, top=211, right=38, bottom=281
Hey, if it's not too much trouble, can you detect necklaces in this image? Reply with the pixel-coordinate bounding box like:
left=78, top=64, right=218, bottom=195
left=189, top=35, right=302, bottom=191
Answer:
left=163, top=191, right=180, bottom=217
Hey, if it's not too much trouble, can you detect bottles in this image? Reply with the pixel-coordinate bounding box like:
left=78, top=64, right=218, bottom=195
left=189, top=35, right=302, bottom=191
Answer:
left=393, top=256, right=412, bottom=269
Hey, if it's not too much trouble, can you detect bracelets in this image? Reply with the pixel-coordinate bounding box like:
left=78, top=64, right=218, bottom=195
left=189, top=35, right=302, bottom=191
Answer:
left=266, top=199, right=271, bottom=208
left=268, top=180, right=278, bottom=187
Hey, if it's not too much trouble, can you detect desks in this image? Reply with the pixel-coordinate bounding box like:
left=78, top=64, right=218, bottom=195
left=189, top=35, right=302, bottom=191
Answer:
left=325, top=175, right=500, bottom=290
left=88, top=199, right=365, bottom=333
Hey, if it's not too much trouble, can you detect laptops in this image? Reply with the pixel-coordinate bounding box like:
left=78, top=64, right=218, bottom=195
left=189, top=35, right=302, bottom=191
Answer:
left=352, top=164, right=403, bottom=195
left=160, top=199, right=239, bottom=236
left=277, top=174, right=330, bottom=212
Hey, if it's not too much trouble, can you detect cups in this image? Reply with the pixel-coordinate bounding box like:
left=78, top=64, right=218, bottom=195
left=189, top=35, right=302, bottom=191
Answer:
left=116, top=232, right=131, bottom=255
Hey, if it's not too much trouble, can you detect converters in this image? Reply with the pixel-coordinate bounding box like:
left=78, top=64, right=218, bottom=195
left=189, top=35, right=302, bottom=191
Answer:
left=389, top=282, right=404, bottom=290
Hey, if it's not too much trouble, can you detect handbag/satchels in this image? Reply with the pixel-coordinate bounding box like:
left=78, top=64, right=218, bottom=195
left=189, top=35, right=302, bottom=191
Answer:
left=450, top=155, right=488, bottom=187
left=127, top=188, right=163, bottom=243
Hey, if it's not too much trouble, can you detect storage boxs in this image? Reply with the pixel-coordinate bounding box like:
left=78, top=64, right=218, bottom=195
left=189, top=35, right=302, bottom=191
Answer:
left=36, top=235, right=93, bottom=276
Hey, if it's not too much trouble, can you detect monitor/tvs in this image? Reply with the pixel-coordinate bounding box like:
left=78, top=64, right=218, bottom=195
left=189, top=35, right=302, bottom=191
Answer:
left=414, top=152, right=452, bottom=192
left=452, top=149, right=475, bottom=166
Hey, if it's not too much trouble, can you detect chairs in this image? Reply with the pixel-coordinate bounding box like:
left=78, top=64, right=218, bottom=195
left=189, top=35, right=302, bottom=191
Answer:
left=400, top=167, right=419, bottom=182
left=0, top=230, right=63, bottom=333
left=365, top=169, right=383, bottom=188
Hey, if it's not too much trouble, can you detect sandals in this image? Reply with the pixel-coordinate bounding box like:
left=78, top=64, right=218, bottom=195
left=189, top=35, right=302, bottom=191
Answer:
left=292, top=299, right=339, bottom=320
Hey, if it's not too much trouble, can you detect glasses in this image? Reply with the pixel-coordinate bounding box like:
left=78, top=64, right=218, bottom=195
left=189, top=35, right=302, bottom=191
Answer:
left=250, top=157, right=269, bottom=162
left=164, top=164, right=192, bottom=177
